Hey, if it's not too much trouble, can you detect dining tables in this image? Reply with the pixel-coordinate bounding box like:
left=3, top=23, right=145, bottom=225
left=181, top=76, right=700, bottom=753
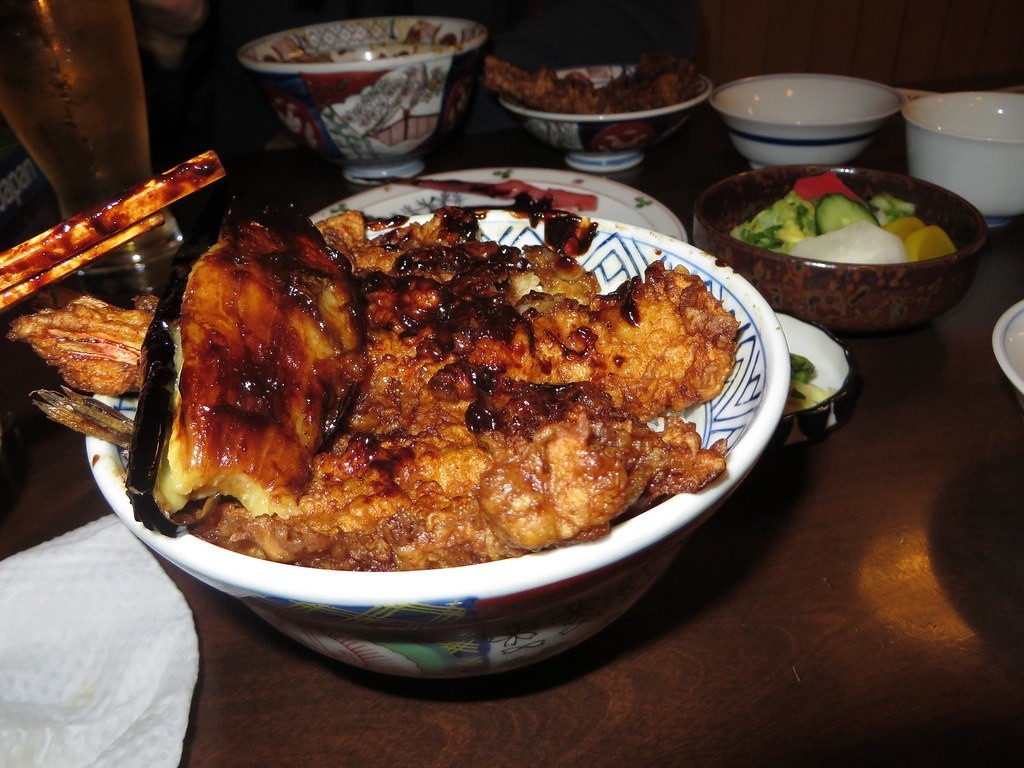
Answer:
left=0, top=73, right=1024, bottom=767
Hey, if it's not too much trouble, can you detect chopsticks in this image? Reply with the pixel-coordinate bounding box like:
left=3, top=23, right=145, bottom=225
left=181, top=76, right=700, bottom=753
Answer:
left=1, top=148, right=227, bottom=311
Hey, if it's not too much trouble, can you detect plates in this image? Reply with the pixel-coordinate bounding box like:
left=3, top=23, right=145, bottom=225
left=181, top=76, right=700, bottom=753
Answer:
left=308, top=168, right=687, bottom=245
left=991, top=298, right=1024, bottom=395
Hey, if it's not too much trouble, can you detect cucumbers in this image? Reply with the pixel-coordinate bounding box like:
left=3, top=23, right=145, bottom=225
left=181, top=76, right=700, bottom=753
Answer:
left=815, top=192, right=881, bottom=236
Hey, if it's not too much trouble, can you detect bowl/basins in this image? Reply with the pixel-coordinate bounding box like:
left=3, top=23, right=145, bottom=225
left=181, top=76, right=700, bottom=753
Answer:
left=691, top=166, right=988, bottom=335
left=899, top=91, right=1024, bottom=228
left=84, top=215, right=791, bottom=681
left=236, top=15, right=487, bottom=180
left=764, top=308, right=861, bottom=447
left=498, top=61, right=713, bottom=173
left=708, top=73, right=905, bottom=167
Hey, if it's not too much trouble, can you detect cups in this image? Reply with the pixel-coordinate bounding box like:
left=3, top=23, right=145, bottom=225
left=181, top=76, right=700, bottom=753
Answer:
left=0, top=0, right=184, bottom=274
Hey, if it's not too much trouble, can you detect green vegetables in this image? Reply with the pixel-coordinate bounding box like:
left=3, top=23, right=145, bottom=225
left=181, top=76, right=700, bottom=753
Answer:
left=728, top=185, right=916, bottom=251
